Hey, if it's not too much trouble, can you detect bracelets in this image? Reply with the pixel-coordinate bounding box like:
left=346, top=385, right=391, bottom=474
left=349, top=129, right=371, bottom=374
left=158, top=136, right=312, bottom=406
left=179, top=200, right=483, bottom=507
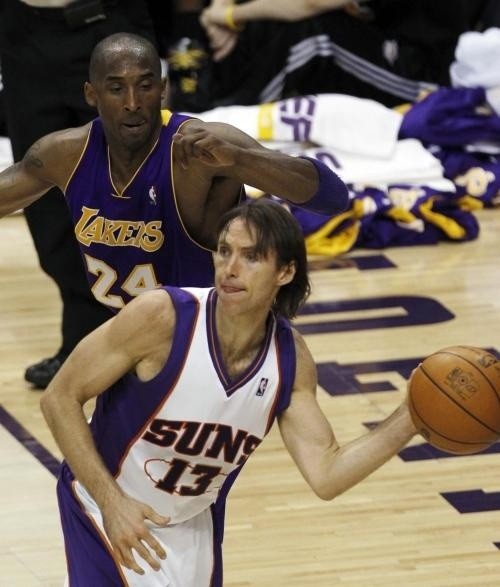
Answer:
left=223, top=4, right=245, bottom=33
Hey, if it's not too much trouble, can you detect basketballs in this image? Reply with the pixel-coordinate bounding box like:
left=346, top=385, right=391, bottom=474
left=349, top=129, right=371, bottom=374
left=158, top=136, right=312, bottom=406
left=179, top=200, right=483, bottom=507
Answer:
left=407, top=345, right=499, bottom=454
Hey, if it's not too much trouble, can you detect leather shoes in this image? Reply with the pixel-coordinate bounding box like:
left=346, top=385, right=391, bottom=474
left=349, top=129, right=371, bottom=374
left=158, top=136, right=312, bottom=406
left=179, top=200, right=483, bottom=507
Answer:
left=25, top=355, right=69, bottom=386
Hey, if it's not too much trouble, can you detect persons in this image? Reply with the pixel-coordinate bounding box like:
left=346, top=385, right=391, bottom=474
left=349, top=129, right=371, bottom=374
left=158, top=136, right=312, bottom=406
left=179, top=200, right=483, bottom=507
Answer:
left=196, top=0, right=461, bottom=108
left=37, top=199, right=424, bottom=586
left=0, top=0, right=157, bottom=392
left=0, top=31, right=348, bottom=316
